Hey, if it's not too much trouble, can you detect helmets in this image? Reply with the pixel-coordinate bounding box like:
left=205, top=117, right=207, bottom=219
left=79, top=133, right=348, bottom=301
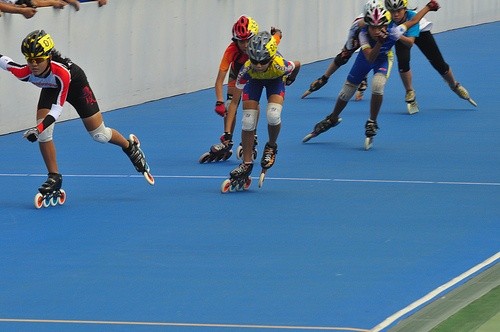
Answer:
left=363, top=7, right=391, bottom=25
left=21, top=30, right=54, bottom=57
left=233, top=16, right=258, bottom=41
left=385, top=0, right=408, bottom=11
left=248, top=31, right=277, bottom=62
left=364, top=0, right=384, bottom=15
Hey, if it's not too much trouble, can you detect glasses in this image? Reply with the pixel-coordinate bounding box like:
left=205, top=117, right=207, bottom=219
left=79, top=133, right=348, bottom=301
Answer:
left=25, top=55, right=50, bottom=63
left=249, top=58, right=272, bottom=65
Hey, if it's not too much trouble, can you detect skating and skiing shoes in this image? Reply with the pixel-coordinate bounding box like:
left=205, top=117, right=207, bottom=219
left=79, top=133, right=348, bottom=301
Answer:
left=237, top=134, right=257, bottom=161
left=221, top=161, right=253, bottom=193
left=199, top=132, right=232, bottom=164
left=453, top=82, right=478, bottom=107
left=405, top=90, right=419, bottom=114
left=356, top=79, right=368, bottom=101
left=259, top=142, right=277, bottom=188
left=34, top=173, right=66, bottom=208
left=302, top=76, right=328, bottom=98
left=303, top=116, right=342, bottom=142
left=365, top=119, right=378, bottom=150
left=122, top=134, right=155, bottom=185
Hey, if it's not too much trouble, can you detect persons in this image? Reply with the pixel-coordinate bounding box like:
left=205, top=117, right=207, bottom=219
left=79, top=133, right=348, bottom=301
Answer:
left=221, top=31, right=301, bottom=192
left=199, top=15, right=283, bottom=164
left=0, top=29, right=154, bottom=208
left=0, top=0, right=107, bottom=19
left=385, top=0, right=478, bottom=115
left=303, top=0, right=440, bottom=150
left=302, top=0, right=383, bottom=101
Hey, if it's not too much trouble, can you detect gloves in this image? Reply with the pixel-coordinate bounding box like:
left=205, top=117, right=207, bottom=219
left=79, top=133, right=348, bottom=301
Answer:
left=215, top=101, right=225, bottom=116
left=427, top=0, right=439, bottom=12
left=24, top=128, right=39, bottom=142
